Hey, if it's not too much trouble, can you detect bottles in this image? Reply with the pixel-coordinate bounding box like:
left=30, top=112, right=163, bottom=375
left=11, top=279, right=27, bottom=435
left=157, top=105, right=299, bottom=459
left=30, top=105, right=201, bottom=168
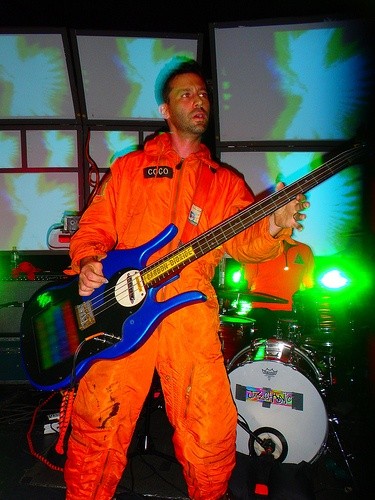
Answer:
left=9, top=247, right=19, bottom=274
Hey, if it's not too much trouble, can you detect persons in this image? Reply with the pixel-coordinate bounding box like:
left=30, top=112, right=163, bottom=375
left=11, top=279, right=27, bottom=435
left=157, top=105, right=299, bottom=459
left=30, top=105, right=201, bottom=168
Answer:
left=64, top=61, right=310, bottom=500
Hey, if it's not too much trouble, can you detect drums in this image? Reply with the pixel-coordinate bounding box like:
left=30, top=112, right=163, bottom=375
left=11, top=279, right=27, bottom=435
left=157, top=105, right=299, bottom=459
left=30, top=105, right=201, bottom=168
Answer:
left=227, top=336, right=331, bottom=464
left=292, top=290, right=357, bottom=349
left=268, top=309, right=305, bottom=341
left=216, top=314, right=256, bottom=367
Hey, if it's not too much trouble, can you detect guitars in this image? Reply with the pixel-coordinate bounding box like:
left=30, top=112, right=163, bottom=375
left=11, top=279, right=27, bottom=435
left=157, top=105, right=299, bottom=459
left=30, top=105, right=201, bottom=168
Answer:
left=16, top=142, right=372, bottom=389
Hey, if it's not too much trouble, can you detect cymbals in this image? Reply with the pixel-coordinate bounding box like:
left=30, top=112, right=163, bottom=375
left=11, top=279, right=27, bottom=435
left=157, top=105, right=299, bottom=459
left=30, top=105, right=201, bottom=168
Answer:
left=217, top=290, right=286, bottom=303
